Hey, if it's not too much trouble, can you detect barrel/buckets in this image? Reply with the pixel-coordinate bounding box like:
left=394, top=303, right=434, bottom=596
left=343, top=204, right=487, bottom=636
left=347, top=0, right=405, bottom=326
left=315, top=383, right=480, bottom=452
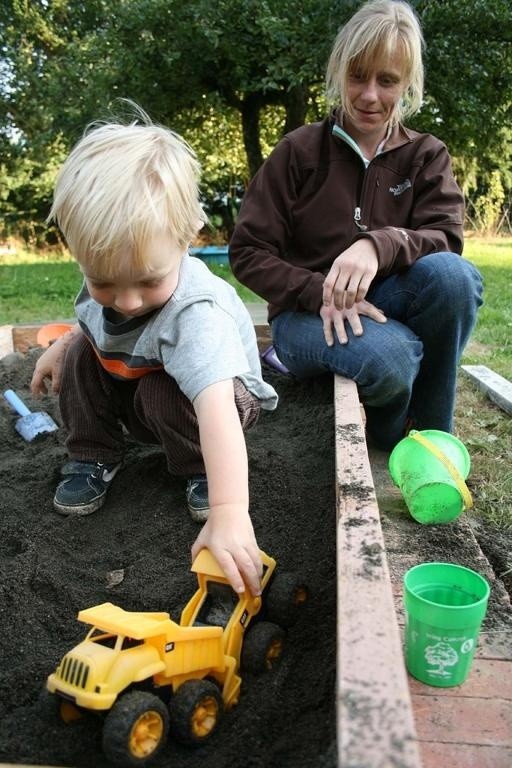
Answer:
left=389, top=428, right=472, bottom=525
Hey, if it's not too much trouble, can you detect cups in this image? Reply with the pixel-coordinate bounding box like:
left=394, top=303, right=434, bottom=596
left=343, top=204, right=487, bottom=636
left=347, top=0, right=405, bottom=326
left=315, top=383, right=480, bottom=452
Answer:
left=397, top=562, right=493, bottom=687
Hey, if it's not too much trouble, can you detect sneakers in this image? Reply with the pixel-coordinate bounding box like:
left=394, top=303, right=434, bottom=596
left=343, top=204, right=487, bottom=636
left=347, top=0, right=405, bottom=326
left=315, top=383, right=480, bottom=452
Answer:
left=53, top=460, right=124, bottom=517
left=186, top=477, right=210, bottom=522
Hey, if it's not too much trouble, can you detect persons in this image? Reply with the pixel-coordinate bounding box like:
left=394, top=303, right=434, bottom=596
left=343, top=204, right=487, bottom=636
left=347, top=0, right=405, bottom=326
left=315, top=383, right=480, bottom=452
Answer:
left=30, top=116, right=285, bottom=602
left=224, top=1, right=487, bottom=447
